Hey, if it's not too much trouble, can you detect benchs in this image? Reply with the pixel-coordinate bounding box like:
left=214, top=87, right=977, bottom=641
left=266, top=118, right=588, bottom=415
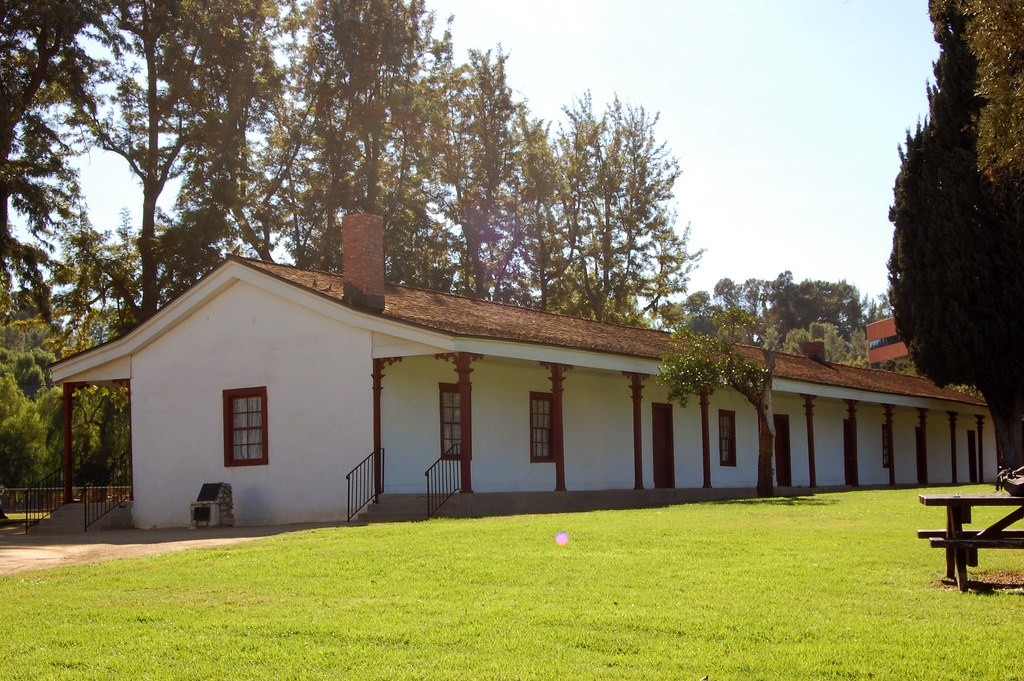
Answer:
left=929, top=536, right=1024, bottom=549
left=916, top=529, right=1024, bottom=539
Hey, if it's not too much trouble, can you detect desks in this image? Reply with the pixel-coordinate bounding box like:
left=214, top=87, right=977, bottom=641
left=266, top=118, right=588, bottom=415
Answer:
left=919, top=493, right=1024, bottom=593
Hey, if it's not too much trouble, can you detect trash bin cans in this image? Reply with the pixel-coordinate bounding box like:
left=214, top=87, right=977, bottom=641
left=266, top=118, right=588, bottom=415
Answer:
left=190, top=481, right=236, bottom=527
